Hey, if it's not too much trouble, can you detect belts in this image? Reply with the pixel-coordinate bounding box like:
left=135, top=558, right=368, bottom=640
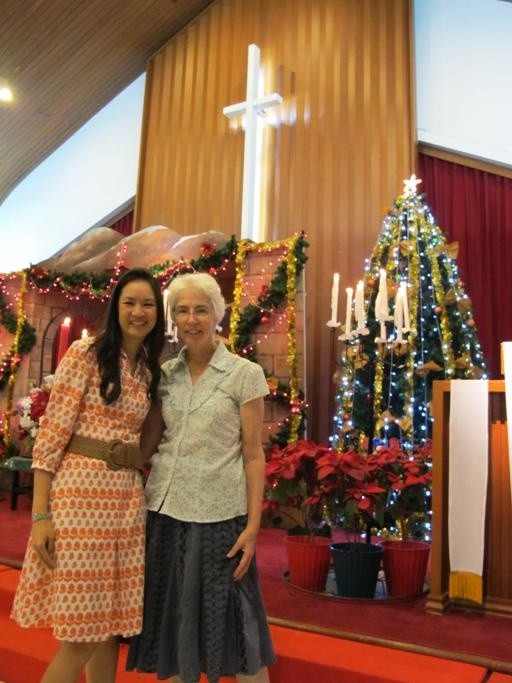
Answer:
left=64, top=433, right=144, bottom=473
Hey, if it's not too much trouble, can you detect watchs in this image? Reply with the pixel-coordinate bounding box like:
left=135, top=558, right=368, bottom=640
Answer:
left=32, top=512, right=49, bottom=521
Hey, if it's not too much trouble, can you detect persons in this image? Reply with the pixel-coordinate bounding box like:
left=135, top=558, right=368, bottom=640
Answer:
left=120, top=272, right=276, bottom=683
left=10, top=266, right=167, bottom=683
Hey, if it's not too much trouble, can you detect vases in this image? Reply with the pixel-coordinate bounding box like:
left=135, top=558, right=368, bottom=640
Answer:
left=284, top=531, right=430, bottom=599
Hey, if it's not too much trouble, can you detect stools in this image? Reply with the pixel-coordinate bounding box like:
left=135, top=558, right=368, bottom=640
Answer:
left=7, top=455, right=39, bottom=512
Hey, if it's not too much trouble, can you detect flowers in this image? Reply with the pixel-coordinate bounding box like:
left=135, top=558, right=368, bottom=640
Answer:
left=265, top=442, right=438, bottom=542
